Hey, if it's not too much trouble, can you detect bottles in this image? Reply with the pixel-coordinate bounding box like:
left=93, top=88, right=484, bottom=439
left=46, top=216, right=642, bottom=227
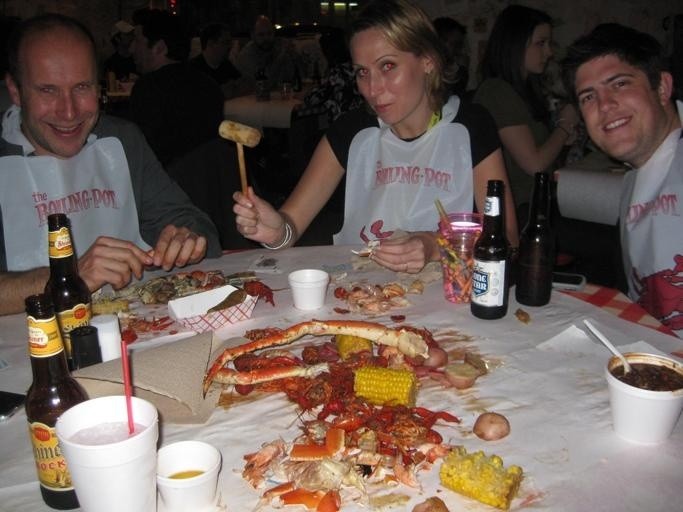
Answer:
left=22, top=294, right=87, bottom=511
left=469, top=180, right=512, bottom=320
left=45, top=211, right=124, bottom=368
left=515, top=169, right=559, bottom=307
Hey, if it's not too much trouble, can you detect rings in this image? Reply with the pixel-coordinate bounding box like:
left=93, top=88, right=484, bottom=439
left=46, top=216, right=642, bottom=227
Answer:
left=405, top=263, right=408, bottom=272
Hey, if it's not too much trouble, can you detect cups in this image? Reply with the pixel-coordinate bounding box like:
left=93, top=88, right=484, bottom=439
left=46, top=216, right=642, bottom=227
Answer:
left=155, top=440, right=223, bottom=510
left=56, top=396, right=159, bottom=511
left=603, top=351, right=682, bottom=449
left=286, top=268, right=332, bottom=311
left=435, top=213, right=485, bottom=305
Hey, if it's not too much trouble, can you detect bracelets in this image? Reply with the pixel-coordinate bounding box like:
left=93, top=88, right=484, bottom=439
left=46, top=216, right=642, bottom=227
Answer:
left=261, top=222, right=293, bottom=250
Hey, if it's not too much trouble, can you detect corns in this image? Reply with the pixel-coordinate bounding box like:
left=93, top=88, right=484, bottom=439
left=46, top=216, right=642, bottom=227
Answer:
left=439, top=445, right=522, bottom=510
left=354, top=367, right=421, bottom=410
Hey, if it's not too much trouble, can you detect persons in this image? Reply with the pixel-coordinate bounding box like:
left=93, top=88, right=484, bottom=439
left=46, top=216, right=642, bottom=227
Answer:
left=566, top=24, right=682, bottom=337
left=104, top=7, right=365, bottom=196
left=1, top=12, right=222, bottom=315
left=435, top=4, right=582, bottom=225
left=233, top=2, right=519, bottom=273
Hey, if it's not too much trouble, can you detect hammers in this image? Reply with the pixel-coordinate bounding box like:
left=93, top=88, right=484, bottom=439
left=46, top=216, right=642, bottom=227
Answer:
left=219, top=120, right=261, bottom=197
